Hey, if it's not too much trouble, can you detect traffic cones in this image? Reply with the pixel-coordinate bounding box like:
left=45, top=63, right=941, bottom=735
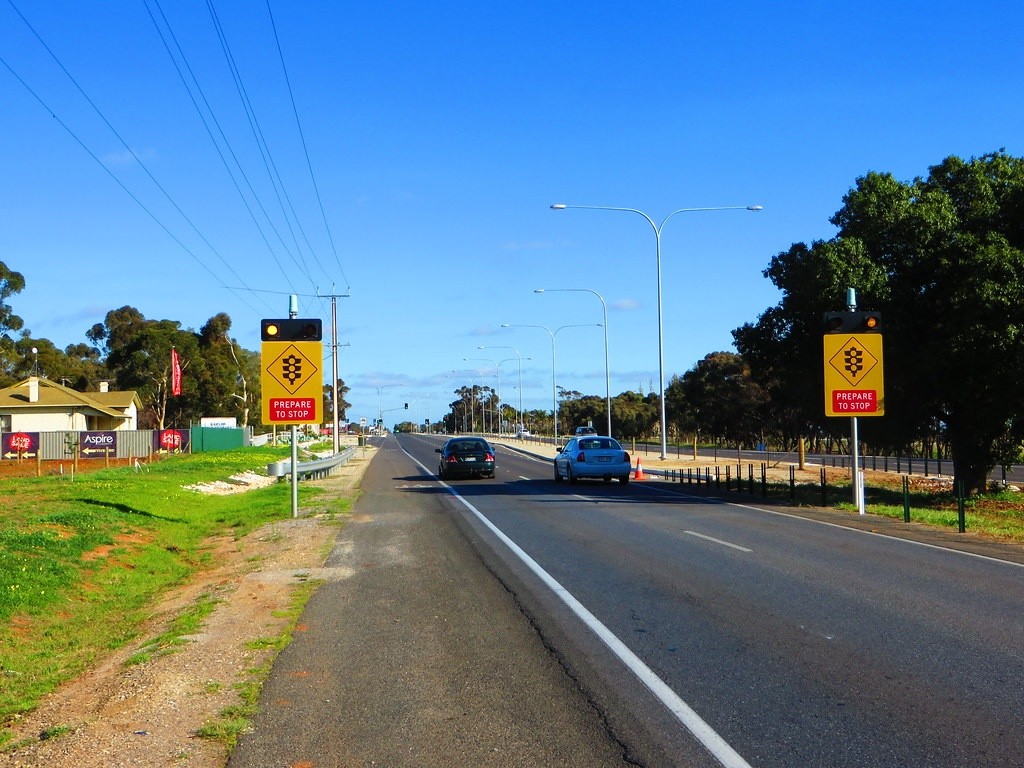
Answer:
left=634, top=457, right=648, bottom=480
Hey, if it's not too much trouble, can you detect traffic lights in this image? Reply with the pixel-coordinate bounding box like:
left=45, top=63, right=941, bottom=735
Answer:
left=261, top=317, right=323, bottom=340
left=379, top=418, right=383, bottom=424
left=425, top=419, right=428, bottom=423
left=824, top=309, right=882, bottom=334
left=405, top=402, right=409, bottom=409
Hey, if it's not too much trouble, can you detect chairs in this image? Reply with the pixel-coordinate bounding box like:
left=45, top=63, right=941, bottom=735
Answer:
left=599, top=440, right=609, bottom=448
left=451, top=444, right=458, bottom=449
left=473, top=443, right=482, bottom=449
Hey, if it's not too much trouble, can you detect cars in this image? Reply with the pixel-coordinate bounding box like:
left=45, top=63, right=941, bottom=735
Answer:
left=554, top=435, right=631, bottom=486
left=518, top=428, right=530, bottom=438
left=435, top=437, right=496, bottom=479
left=395, top=429, right=399, bottom=433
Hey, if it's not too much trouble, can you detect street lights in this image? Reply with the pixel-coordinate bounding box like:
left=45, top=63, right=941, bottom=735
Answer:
left=535, top=290, right=612, bottom=441
left=462, top=356, right=533, bottom=438
left=501, top=324, right=605, bottom=445
left=547, top=203, right=765, bottom=459
left=452, top=369, right=486, bottom=437
left=477, top=345, right=524, bottom=442
left=445, top=374, right=498, bottom=435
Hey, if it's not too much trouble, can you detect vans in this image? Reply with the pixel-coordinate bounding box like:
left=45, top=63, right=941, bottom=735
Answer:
left=575, top=426, right=599, bottom=443
